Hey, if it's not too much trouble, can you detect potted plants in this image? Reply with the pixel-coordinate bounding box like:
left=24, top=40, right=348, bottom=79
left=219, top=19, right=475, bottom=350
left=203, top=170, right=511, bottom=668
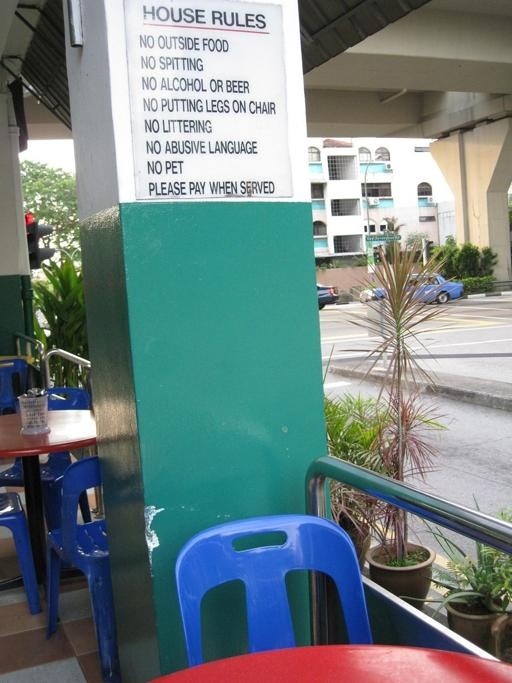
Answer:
left=337, top=230, right=464, bottom=610
left=397, top=494, right=504, bottom=657
left=430, top=509, right=512, bottom=662
left=322, top=392, right=443, bottom=573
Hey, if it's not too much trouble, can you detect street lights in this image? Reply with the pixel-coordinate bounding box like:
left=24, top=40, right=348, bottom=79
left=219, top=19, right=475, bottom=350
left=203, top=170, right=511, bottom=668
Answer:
left=363, top=152, right=383, bottom=274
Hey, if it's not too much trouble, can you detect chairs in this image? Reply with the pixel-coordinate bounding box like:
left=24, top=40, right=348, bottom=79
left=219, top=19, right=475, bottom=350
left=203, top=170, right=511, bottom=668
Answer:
left=0, top=492, right=42, bottom=614
left=175, top=516, right=378, bottom=668
left=1, top=358, right=27, bottom=414
left=1, top=388, right=91, bottom=569
left=46, top=455, right=119, bottom=683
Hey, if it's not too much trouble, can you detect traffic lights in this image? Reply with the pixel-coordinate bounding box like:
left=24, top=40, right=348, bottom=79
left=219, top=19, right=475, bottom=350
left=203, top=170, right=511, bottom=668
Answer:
left=23, top=212, right=56, bottom=269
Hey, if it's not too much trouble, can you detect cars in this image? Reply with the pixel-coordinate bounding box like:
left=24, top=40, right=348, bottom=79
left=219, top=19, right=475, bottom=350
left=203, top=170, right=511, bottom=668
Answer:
left=359, top=272, right=465, bottom=305
left=316, top=282, right=339, bottom=310
